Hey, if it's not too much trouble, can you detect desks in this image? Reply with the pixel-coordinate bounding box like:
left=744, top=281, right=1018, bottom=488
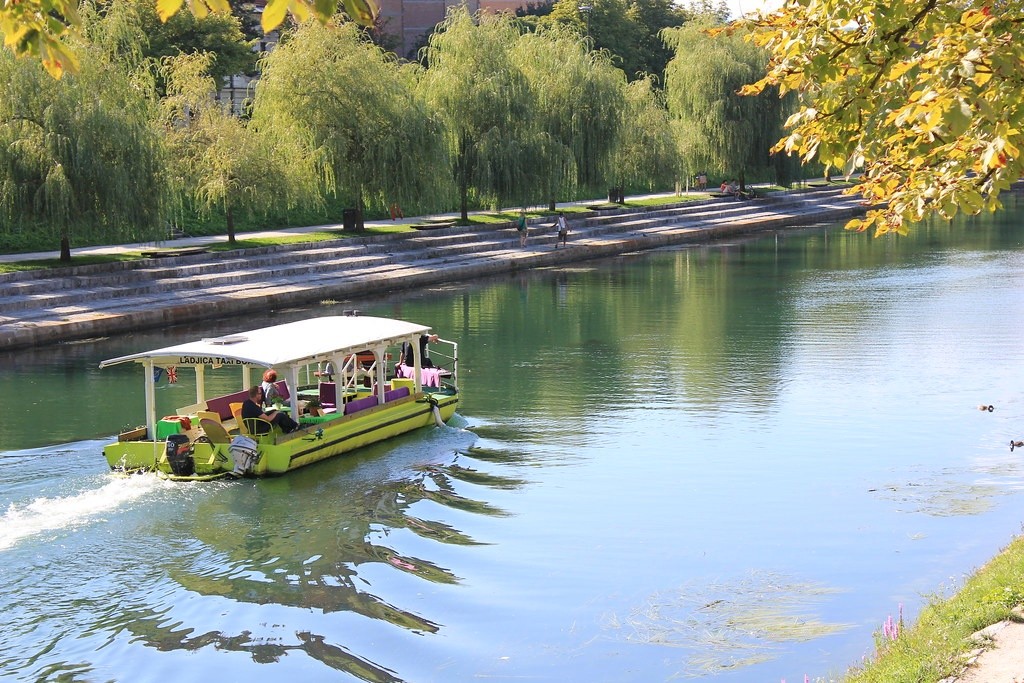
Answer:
left=299, top=413, right=343, bottom=429
left=157, top=415, right=200, bottom=440
left=264, top=406, right=292, bottom=417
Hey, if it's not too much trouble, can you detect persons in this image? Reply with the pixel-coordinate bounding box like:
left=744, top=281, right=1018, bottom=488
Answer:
left=396, top=334, right=438, bottom=369
left=721, top=180, right=734, bottom=193
left=550, top=212, right=571, bottom=248
left=731, top=179, right=740, bottom=201
left=519, top=212, right=528, bottom=248
left=242, top=386, right=300, bottom=434
left=695, top=171, right=708, bottom=191
left=263, top=369, right=290, bottom=407
left=326, top=362, right=360, bottom=387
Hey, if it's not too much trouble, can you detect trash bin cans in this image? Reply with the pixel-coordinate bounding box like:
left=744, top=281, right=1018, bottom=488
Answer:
left=342, top=209, right=356, bottom=231
left=607, top=187, right=618, bottom=202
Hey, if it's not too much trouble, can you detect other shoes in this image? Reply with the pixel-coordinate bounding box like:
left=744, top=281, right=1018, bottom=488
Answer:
left=555, top=245, right=557, bottom=248
left=735, top=198, right=740, bottom=202
left=521, top=244, right=526, bottom=249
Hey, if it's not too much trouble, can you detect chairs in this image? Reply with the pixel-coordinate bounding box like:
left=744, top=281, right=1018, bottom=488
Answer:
left=197, top=376, right=416, bottom=446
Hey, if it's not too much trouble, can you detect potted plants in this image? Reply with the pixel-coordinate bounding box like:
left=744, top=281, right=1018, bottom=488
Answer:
left=305, top=397, right=322, bottom=416
left=271, top=395, right=284, bottom=409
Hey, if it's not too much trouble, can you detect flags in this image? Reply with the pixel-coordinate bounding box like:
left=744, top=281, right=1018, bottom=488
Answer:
left=154, top=366, right=163, bottom=382
left=167, top=367, right=177, bottom=383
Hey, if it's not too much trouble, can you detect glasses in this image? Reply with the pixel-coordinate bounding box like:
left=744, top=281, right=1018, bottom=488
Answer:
left=258, top=392, right=262, bottom=395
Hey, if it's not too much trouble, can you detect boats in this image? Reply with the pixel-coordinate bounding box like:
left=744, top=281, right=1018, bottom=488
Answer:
left=100, top=312, right=460, bottom=481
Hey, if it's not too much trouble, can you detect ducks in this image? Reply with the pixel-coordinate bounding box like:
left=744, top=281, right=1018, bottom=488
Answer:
left=977, top=404, right=995, bottom=412
left=1010, top=440, right=1024, bottom=447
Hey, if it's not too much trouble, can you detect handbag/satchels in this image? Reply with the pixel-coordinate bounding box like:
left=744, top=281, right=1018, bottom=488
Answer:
left=517, top=225, right=523, bottom=231
left=561, top=227, right=567, bottom=234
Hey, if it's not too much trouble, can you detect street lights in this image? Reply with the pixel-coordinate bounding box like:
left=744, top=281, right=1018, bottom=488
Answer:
left=577, top=7, right=591, bottom=53
